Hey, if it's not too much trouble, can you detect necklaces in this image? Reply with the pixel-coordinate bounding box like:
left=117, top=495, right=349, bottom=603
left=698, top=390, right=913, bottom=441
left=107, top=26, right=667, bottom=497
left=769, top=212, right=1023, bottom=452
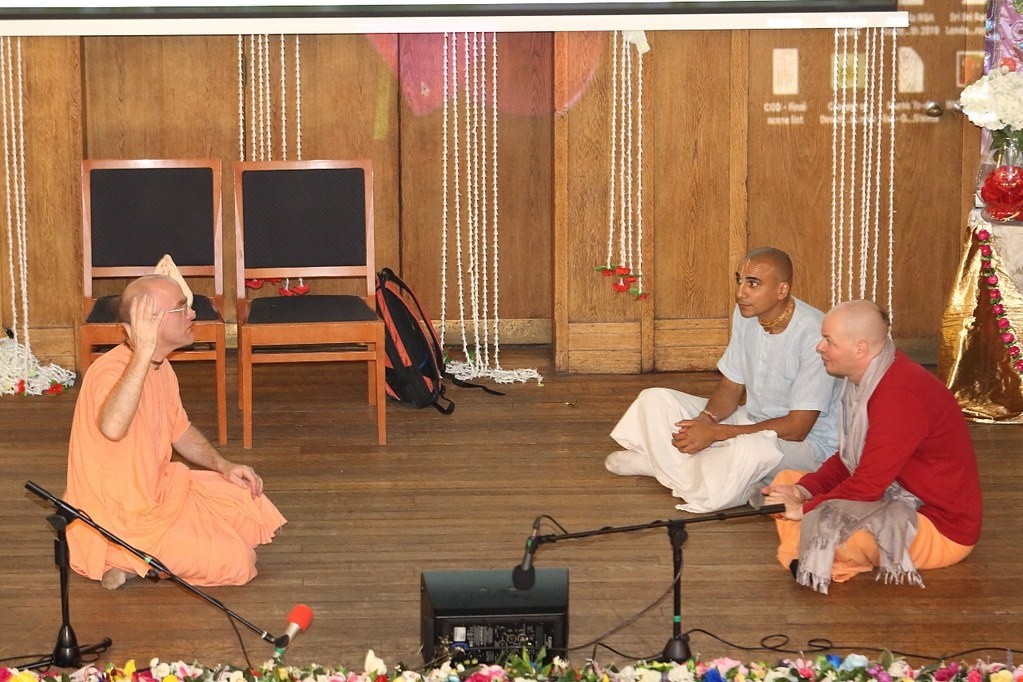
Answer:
left=125, top=343, right=163, bottom=365
left=758, top=297, right=796, bottom=334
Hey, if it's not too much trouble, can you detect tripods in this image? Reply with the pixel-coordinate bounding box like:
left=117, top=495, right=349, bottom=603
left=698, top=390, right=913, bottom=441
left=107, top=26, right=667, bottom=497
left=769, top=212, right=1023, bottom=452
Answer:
left=14, top=480, right=275, bottom=671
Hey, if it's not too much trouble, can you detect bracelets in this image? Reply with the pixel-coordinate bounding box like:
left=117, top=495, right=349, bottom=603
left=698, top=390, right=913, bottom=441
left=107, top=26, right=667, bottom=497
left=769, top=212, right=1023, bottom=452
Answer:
left=703, top=409, right=719, bottom=424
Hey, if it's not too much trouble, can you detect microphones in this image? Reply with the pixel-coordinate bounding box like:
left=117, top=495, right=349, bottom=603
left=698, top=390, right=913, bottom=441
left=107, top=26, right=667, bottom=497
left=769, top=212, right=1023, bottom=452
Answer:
left=513, top=516, right=541, bottom=590
left=271, top=604, right=312, bottom=664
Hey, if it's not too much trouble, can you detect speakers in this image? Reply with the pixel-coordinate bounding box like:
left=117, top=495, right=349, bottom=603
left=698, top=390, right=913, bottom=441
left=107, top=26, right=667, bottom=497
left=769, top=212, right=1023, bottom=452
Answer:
left=419, top=567, right=569, bottom=671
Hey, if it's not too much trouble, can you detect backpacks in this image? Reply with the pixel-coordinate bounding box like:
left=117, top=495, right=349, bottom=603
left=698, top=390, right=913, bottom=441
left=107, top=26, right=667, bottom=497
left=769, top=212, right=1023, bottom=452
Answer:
left=375, top=267, right=455, bottom=415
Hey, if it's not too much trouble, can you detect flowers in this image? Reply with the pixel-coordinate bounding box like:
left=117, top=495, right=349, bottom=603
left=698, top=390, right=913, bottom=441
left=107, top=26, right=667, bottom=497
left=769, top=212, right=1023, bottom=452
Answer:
left=1, top=647, right=1023, bottom=682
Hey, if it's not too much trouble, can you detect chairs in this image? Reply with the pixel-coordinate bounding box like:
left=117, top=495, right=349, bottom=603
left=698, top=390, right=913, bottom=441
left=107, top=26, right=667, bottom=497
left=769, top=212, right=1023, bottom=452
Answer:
left=75, top=157, right=227, bottom=447
left=231, top=159, right=387, bottom=452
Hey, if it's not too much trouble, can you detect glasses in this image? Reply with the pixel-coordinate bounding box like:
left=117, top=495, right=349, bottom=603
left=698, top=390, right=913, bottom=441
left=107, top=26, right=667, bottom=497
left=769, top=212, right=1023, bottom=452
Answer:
left=152, top=303, right=187, bottom=317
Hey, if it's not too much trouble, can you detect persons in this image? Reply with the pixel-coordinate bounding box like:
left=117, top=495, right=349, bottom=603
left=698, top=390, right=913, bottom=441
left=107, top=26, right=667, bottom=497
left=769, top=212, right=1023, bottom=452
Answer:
left=759, top=300, right=985, bottom=595
left=63, top=275, right=288, bottom=590
left=605, top=245, right=849, bottom=512
